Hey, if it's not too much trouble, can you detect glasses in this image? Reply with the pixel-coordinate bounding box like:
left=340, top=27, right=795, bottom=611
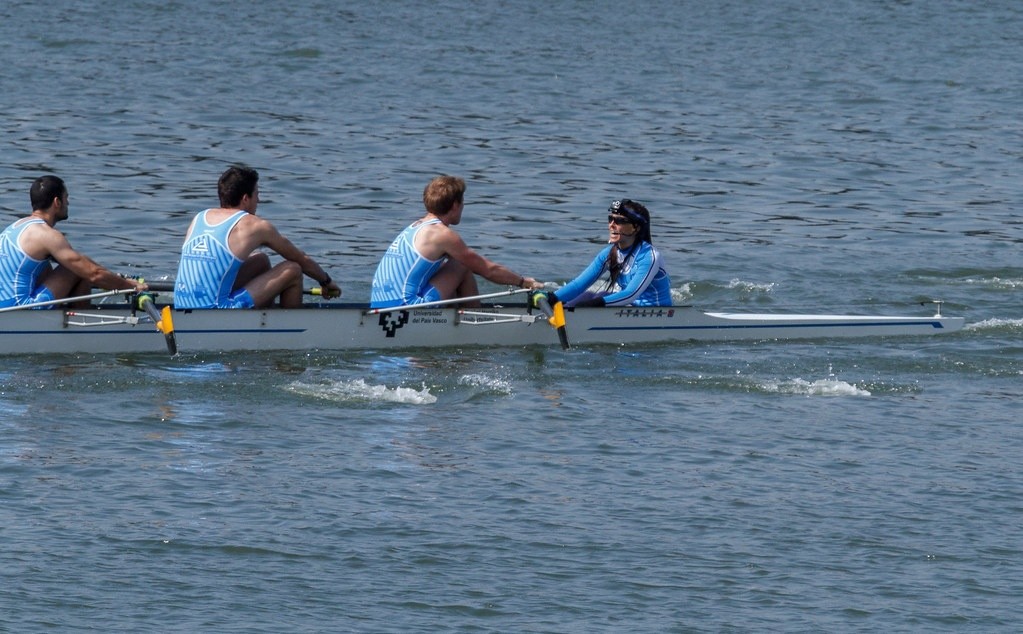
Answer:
left=609, top=214, right=633, bottom=224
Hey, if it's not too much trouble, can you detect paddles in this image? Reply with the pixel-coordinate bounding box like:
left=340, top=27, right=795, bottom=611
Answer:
left=118, top=273, right=341, bottom=298
left=135, top=292, right=180, bottom=358
left=531, top=291, right=570, bottom=352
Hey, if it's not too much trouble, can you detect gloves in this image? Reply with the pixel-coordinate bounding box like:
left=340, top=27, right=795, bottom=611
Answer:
left=533, top=290, right=549, bottom=307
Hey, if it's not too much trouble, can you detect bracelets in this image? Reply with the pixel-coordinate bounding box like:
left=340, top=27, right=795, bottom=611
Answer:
left=317, top=272, right=333, bottom=288
left=516, top=277, right=524, bottom=287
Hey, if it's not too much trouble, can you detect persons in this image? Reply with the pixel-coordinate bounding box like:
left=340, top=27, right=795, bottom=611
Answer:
left=528, top=198, right=673, bottom=308
left=172, top=166, right=342, bottom=310
left=370, top=177, right=544, bottom=308
left=0, top=175, right=149, bottom=312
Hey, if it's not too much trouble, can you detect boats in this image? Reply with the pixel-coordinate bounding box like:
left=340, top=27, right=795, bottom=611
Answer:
left=0, top=302, right=969, bottom=357
